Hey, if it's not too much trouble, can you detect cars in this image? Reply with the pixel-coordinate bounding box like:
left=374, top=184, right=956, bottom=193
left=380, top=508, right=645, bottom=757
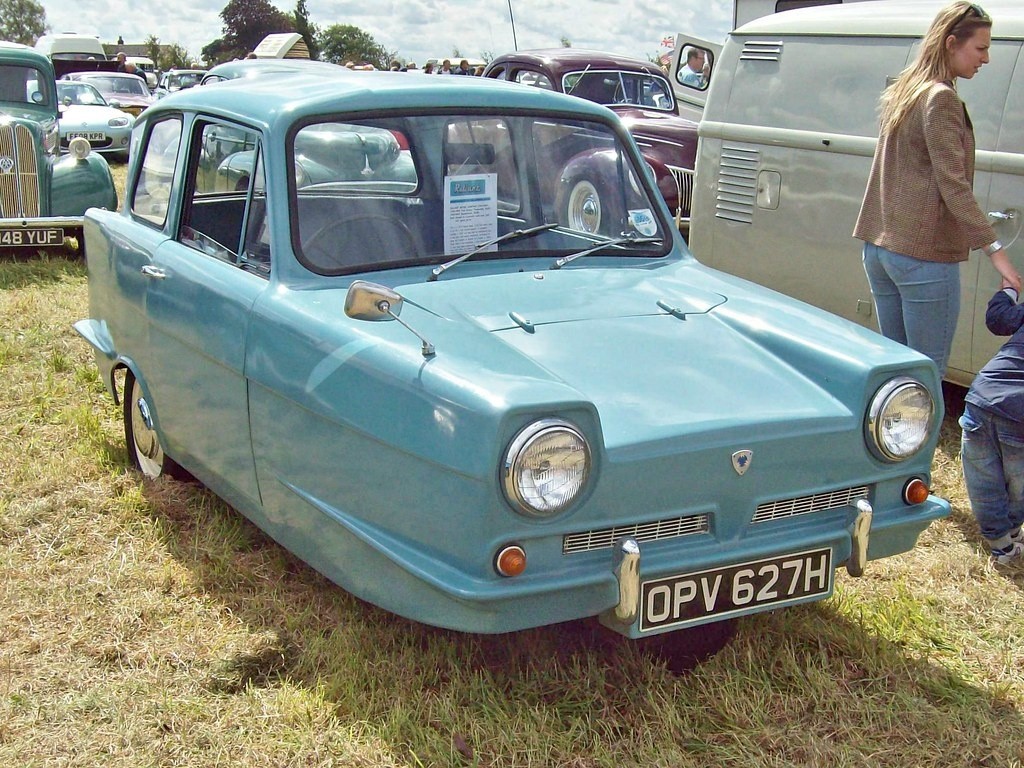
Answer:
left=1, top=2, right=1024, bottom=397
left=68, top=67, right=951, bottom=636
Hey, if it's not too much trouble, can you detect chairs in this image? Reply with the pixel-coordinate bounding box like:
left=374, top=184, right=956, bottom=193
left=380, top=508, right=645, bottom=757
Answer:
left=258, top=198, right=423, bottom=260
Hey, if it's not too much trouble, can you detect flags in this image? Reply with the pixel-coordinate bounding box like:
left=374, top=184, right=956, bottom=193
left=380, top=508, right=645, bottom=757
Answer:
left=659, top=36, right=674, bottom=49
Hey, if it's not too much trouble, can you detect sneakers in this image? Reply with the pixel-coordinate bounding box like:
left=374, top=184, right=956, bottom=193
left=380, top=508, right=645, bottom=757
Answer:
left=1010, top=527, right=1024, bottom=543
left=990, top=542, right=1024, bottom=564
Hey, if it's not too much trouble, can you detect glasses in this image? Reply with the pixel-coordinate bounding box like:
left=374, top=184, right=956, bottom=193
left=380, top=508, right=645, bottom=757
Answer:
left=948, top=4, right=984, bottom=33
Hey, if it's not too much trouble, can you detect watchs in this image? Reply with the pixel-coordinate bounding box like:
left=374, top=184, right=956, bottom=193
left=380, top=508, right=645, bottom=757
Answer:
left=984, top=240, right=1004, bottom=257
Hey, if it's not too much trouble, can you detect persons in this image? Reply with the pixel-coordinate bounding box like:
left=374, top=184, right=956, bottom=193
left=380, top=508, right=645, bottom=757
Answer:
left=169, top=52, right=257, bottom=72
left=87, top=52, right=148, bottom=94
left=959, top=274, right=1024, bottom=564
left=345, top=55, right=485, bottom=76
left=853, top=0, right=1021, bottom=379
left=676, top=48, right=710, bottom=88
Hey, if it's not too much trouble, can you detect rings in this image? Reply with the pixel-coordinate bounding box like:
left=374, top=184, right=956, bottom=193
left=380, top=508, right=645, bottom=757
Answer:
left=1018, top=275, right=1021, bottom=279
